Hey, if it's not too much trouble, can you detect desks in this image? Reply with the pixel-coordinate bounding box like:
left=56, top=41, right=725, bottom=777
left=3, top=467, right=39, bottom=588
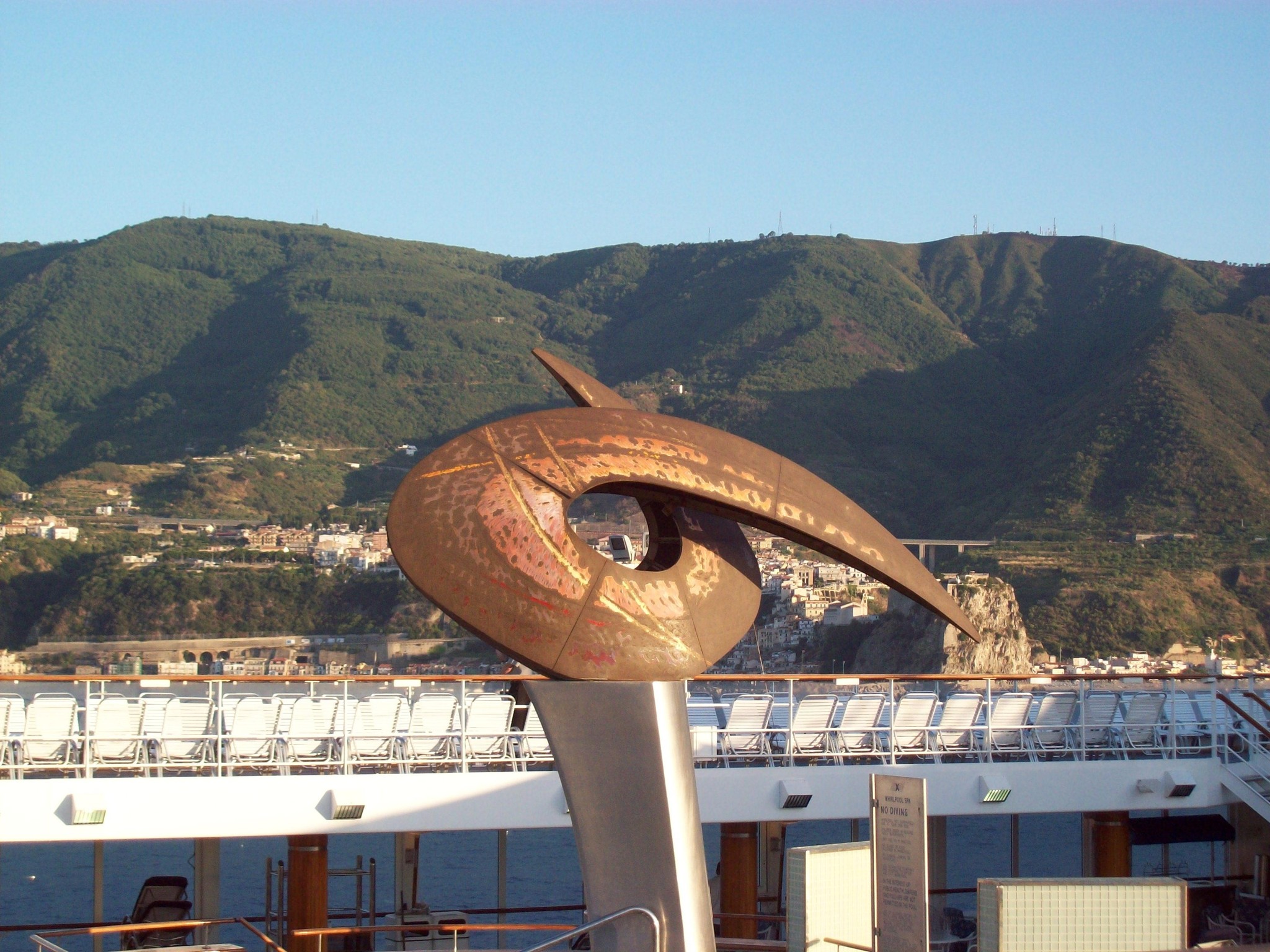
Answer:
left=930, top=930, right=960, bottom=952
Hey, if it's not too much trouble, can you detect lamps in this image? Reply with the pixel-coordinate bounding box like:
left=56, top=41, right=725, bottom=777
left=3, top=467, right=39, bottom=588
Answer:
left=330, top=789, right=366, bottom=820
left=979, top=776, right=1011, bottom=804
left=70, top=793, right=106, bottom=824
left=779, top=779, right=814, bottom=809
left=1164, top=770, right=1197, bottom=798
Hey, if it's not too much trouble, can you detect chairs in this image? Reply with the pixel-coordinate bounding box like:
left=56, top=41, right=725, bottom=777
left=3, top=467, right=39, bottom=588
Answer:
left=1205, top=913, right=1259, bottom=945
left=120, top=875, right=192, bottom=951
left=930, top=905, right=976, bottom=952
left=0, top=690, right=1270, bottom=779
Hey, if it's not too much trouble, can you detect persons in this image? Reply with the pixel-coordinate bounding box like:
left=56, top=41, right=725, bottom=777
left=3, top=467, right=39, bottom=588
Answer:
left=708, top=862, right=723, bottom=937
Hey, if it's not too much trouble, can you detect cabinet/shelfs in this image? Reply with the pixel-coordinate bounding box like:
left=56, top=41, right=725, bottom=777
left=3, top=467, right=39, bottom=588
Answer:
left=264, top=854, right=377, bottom=952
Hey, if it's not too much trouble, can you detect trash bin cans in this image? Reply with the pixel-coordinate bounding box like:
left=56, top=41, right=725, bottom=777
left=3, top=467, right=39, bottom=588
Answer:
left=385, top=914, right=433, bottom=950
left=429, top=911, right=469, bottom=950
